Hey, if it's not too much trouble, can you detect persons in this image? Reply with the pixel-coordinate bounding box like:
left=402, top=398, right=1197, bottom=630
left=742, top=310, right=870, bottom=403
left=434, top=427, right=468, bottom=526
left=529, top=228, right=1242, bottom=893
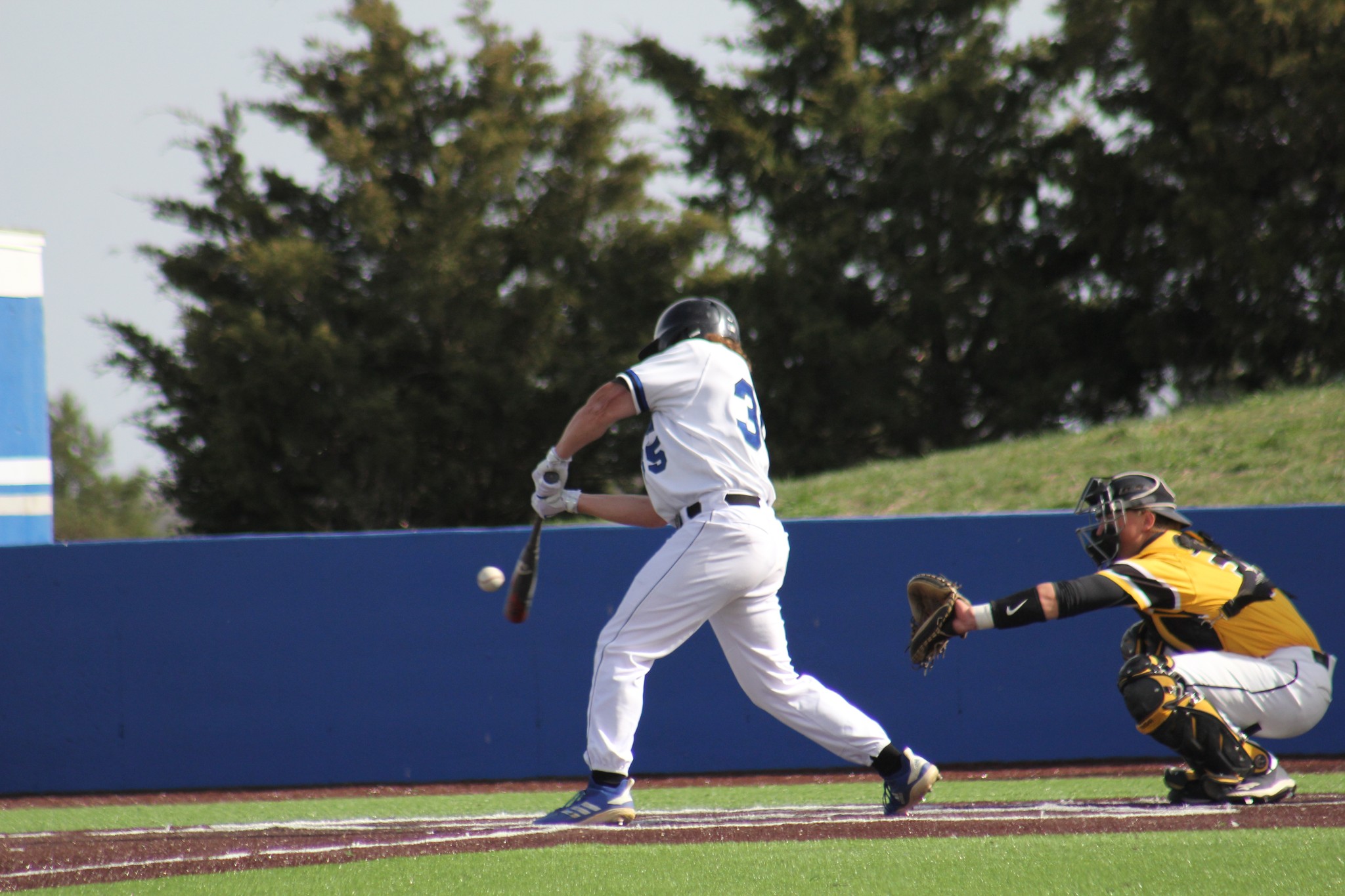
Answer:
left=947, top=473, right=1337, bottom=804
left=530, top=298, right=945, bottom=827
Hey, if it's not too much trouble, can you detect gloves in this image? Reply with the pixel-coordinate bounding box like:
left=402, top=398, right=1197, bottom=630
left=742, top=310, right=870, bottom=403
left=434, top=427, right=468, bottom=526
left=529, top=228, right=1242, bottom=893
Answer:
left=531, top=489, right=581, bottom=521
left=531, top=446, right=573, bottom=496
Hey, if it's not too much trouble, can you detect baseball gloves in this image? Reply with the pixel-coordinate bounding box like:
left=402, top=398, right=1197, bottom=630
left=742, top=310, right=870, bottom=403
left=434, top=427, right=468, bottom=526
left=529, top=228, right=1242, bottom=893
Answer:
left=904, top=572, right=971, bottom=672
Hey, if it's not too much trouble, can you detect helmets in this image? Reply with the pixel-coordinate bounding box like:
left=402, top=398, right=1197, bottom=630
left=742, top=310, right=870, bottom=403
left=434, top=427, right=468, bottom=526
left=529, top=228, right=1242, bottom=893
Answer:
left=1083, top=472, right=1192, bottom=528
left=637, top=298, right=741, bottom=362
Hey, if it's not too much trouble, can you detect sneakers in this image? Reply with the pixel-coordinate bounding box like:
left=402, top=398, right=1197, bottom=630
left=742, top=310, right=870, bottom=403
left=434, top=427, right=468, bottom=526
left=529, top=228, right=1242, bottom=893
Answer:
left=1163, top=765, right=1243, bottom=804
left=532, top=775, right=636, bottom=825
left=882, top=746, right=942, bottom=816
left=1225, top=764, right=1297, bottom=803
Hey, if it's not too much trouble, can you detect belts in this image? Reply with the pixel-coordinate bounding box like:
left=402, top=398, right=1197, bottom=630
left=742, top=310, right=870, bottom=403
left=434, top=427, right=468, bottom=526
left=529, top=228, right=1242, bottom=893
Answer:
left=674, top=494, right=759, bottom=529
left=1312, top=650, right=1329, bottom=669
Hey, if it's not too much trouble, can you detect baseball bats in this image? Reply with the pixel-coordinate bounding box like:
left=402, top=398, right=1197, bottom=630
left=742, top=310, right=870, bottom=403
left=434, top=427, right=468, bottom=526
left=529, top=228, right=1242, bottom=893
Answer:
left=502, top=470, right=560, bottom=625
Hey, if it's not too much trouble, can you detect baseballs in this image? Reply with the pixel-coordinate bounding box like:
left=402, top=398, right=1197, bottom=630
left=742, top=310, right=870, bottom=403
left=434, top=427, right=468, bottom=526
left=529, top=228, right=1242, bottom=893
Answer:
left=476, top=565, right=505, bottom=593
